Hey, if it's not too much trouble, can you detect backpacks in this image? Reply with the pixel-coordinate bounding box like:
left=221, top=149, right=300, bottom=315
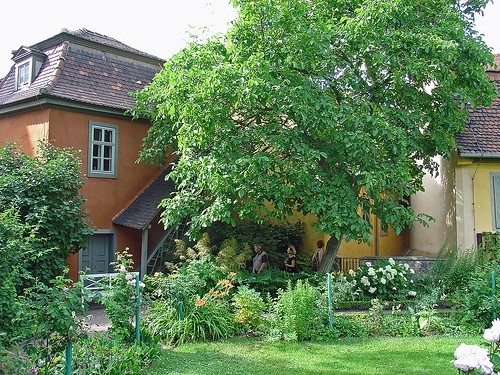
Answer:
left=312, top=255, right=317, bottom=272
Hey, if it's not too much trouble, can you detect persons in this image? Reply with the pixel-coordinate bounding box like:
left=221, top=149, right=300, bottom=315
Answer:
left=312, top=240, right=325, bottom=275
left=283, top=245, right=297, bottom=273
left=251, top=244, right=271, bottom=274
